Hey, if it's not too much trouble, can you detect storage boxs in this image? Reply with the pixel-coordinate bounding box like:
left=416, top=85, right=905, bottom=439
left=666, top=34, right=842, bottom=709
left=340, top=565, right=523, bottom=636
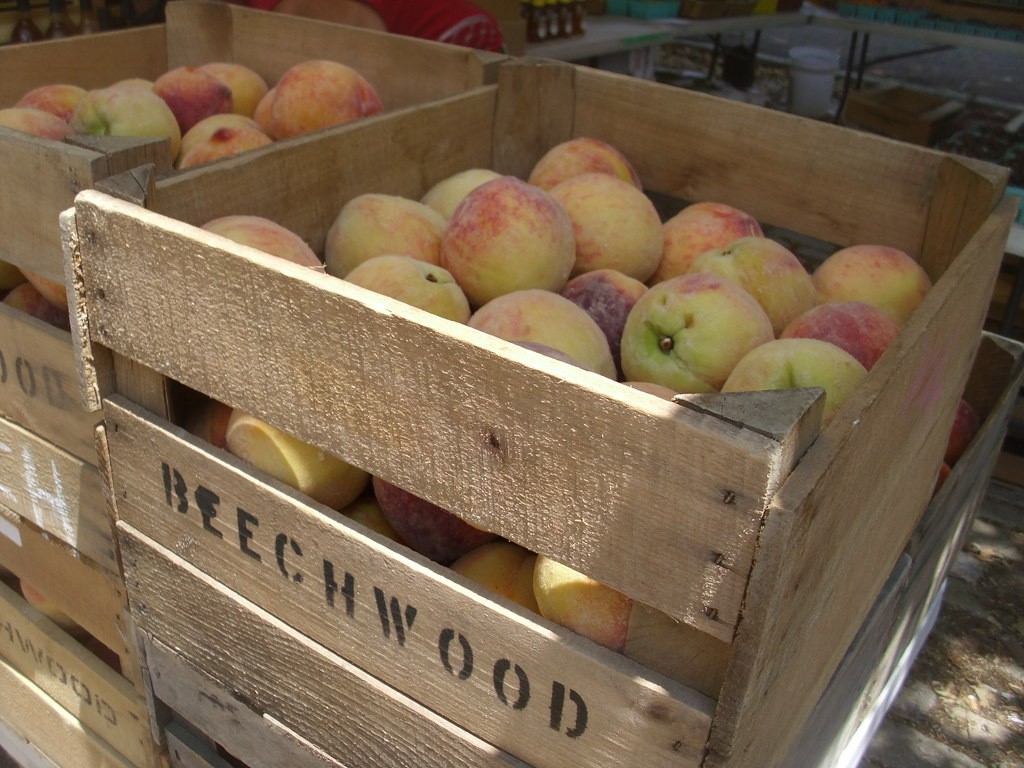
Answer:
left=0, top=0, right=1024, bottom=767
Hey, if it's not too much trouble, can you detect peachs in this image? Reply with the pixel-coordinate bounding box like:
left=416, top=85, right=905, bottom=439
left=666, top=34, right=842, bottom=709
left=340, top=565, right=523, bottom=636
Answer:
left=0, top=62, right=983, bottom=675
